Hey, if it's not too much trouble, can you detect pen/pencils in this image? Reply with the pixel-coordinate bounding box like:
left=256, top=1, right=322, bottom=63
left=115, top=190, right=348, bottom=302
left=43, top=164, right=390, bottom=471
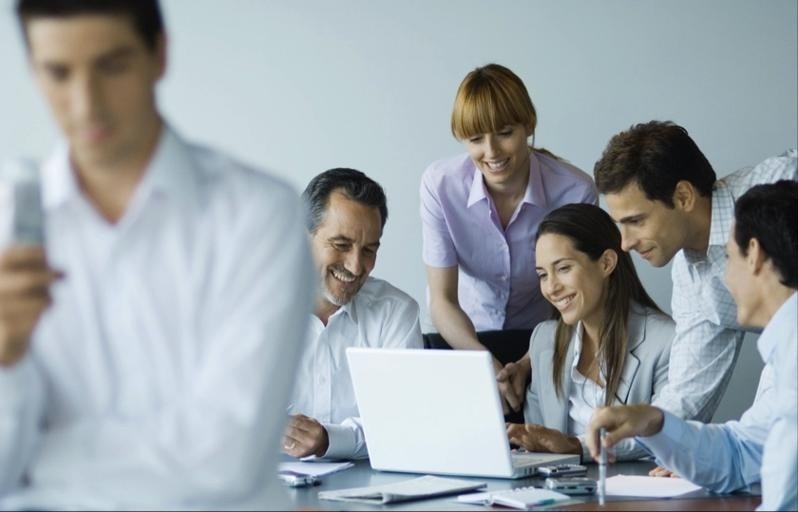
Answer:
left=596, top=427, right=606, bottom=505
left=285, top=404, right=293, bottom=412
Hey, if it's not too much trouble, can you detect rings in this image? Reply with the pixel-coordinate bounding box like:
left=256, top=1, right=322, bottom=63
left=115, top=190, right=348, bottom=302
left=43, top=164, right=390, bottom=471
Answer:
left=284, top=441, right=296, bottom=451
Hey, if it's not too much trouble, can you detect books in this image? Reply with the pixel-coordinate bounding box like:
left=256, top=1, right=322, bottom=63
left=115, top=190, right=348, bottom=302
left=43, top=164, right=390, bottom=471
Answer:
left=455, top=486, right=584, bottom=510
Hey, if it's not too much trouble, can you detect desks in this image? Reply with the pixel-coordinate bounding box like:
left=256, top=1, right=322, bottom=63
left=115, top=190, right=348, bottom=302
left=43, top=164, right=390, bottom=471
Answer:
left=272, top=450, right=763, bottom=511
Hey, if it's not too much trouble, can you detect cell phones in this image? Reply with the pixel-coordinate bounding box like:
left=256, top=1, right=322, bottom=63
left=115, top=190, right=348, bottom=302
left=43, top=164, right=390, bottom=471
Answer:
left=545, top=477, right=597, bottom=494
left=15, top=184, right=43, bottom=246
left=538, top=464, right=587, bottom=477
left=278, top=471, right=312, bottom=487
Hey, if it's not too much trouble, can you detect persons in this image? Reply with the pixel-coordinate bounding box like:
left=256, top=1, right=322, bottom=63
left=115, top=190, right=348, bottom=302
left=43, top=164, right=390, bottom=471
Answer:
left=521, top=117, right=798, bottom=477
left=0, top=0, right=317, bottom=510
left=274, top=164, right=426, bottom=458
left=586, top=178, right=798, bottom=510
left=501, top=209, right=675, bottom=457
left=422, top=61, right=600, bottom=416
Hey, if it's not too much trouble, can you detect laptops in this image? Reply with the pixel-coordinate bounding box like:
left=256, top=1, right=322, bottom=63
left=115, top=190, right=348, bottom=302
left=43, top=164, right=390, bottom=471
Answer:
left=346, top=347, right=581, bottom=479
